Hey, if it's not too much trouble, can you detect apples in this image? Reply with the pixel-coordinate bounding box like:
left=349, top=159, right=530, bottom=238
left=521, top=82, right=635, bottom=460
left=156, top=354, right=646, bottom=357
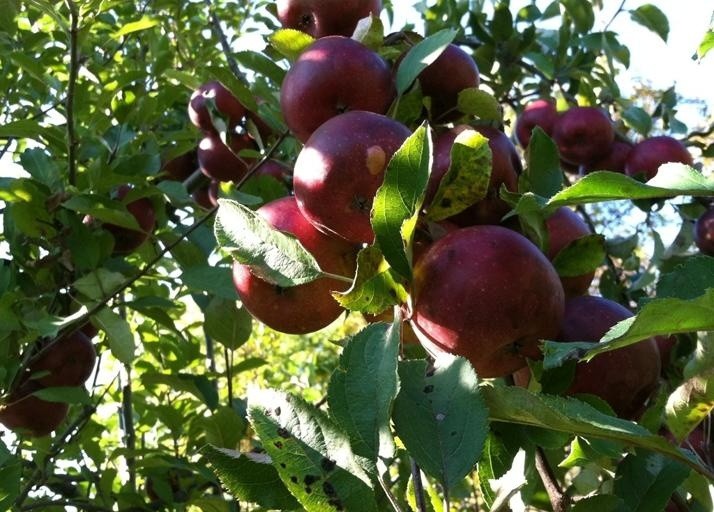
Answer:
left=0, top=0, right=714, bottom=512
